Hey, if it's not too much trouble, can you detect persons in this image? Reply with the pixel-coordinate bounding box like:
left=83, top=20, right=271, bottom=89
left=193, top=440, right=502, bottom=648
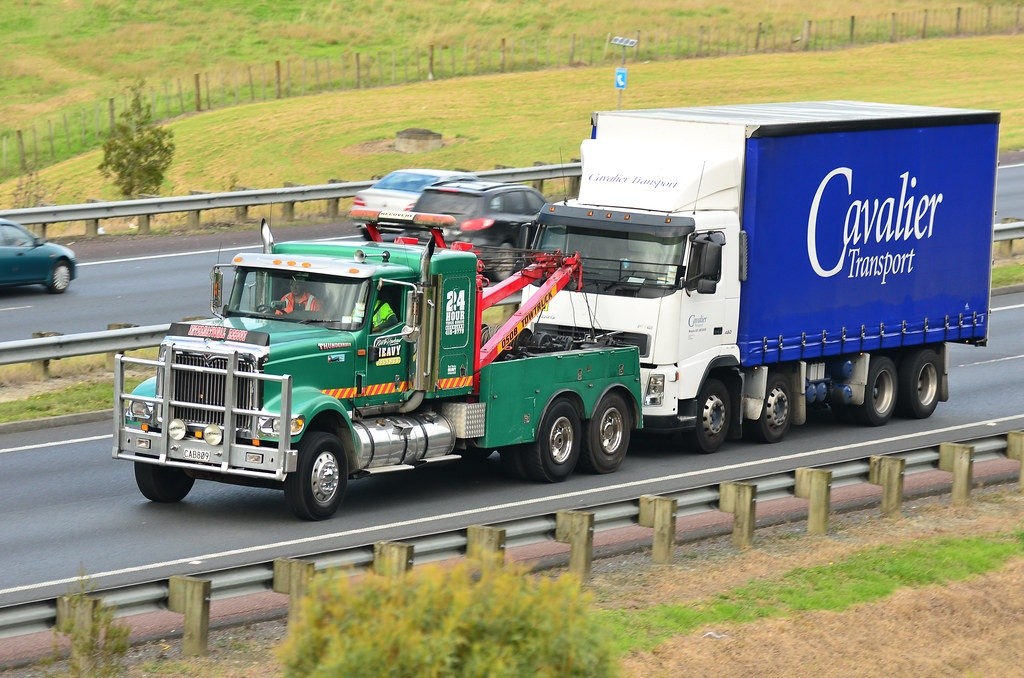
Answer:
left=346, top=285, right=398, bottom=333
left=275, top=279, right=324, bottom=315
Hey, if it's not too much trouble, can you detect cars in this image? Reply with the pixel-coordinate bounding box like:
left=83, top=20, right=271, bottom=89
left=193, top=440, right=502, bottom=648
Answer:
left=0, top=218, right=78, bottom=294
left=347, top=168, right=476, bottom=241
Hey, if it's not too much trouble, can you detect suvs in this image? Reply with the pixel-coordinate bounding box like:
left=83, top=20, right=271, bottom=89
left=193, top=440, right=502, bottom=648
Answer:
left=403, top=178, right=548, bottom=283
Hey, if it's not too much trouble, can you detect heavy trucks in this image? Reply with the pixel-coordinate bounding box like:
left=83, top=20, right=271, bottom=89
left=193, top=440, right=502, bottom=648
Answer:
left=109, top=209, right=644, bottom=522
left=518, top=99, right=1001, bottom=454
